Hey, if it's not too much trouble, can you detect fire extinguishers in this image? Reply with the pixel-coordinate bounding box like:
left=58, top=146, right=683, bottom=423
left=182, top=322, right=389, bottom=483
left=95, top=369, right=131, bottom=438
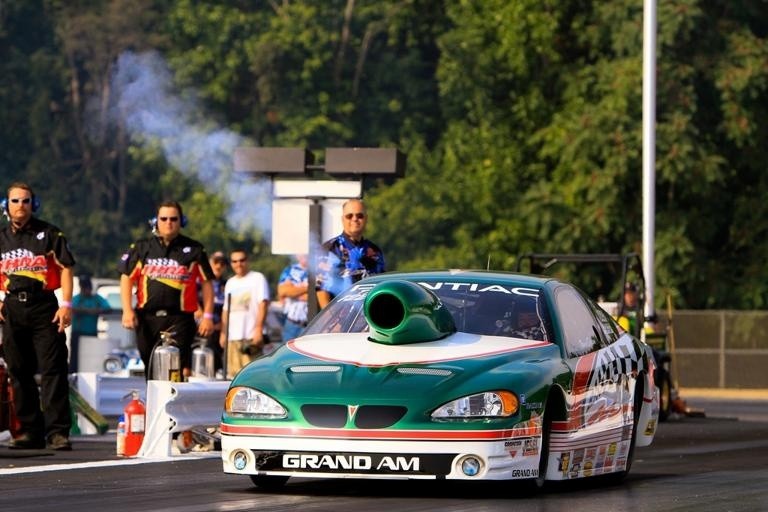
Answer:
left=120, top=389, right=146, bottom=456
left=191, top=337, right=214, bottom=381
left=148, top=325, right=181, bottom=382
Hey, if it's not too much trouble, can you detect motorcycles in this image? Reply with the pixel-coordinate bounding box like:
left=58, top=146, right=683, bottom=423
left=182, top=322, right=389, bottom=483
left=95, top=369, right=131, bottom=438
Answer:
left=611, top=314, right=674, bottom=424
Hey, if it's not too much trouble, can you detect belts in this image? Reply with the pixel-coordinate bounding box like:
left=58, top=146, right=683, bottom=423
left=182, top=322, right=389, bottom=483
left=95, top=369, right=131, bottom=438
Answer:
left=288, top=318, right=308, bottom=328
left=139, top=309, right=182, bottom=317
left=10, top=290, right=48, bottom=302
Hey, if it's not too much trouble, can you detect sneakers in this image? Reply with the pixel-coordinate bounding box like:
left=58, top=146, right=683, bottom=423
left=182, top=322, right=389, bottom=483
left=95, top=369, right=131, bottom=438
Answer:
left=48, top=433, right=72, bottom=450
left=8, top=431, right=46, bottom=449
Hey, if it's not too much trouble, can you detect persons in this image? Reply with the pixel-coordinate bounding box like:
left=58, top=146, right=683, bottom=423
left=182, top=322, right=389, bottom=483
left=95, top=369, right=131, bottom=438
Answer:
left=1, top=178, right=77, bottom=453
left=312, top=196, right=385, bottom=311
left=66, top=274, right=112, bottom=377
left=276, top=245, right=326, bottom=346
left=217, top=248, right=271, bottom=380
left=499, top=300, right=550, bottom=343
left=192, top=249, right=231, bottom=375
left=613, top=282, right=688, bottom=416
left=108, top=198, right=216, bottom=383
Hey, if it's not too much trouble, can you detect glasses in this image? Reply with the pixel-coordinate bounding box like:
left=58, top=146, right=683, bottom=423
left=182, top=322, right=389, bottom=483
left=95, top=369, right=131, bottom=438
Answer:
left=343, top=214, right=365, bottom=219
left=212, top=256, right=226, bottom=263
left=231, top=258, right=246, bottom=263
left=159, top=217, right=178, bottom=221
left=10, top=198, right=31, bottom=203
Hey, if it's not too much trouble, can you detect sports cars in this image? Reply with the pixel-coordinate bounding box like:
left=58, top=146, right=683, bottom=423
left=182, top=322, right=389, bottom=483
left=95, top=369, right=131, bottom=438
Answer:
left=219, top=269, right=661, bottom=489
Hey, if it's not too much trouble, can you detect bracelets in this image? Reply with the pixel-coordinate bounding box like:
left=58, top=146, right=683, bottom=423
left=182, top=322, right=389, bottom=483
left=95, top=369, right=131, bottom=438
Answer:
left=61, top=301, right=73, bottom=310
left=203, top=312, right=214, bottom=321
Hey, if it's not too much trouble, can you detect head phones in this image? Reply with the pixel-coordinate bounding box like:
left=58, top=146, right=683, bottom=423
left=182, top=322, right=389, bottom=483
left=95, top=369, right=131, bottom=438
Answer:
left=0, top=196, right=40, bottom=212
left=150, top=216, right=188, bottom=228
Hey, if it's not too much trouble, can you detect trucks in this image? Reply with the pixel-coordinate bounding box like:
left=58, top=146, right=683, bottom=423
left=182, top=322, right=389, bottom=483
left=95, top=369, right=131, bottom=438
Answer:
left=54, top=277, right=138, bottom=368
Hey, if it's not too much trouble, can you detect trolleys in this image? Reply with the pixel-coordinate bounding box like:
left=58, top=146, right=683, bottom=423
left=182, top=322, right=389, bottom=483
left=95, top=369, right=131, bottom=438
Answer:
left=147, top=379, right=232, bottom=454
